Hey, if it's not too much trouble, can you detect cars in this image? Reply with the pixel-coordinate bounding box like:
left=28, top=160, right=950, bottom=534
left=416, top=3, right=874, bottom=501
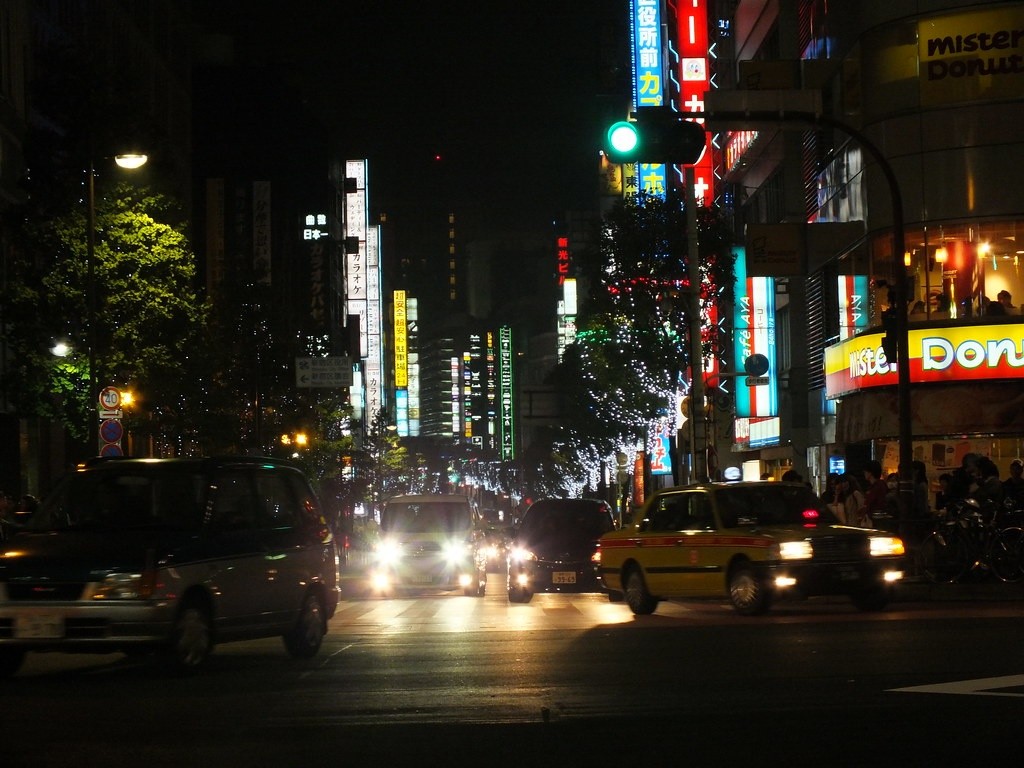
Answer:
left=595, top=479, right=907, bottom=619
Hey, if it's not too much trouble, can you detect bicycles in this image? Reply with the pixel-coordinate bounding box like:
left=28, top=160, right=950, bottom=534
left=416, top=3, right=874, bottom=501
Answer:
left=912, top=499, right=1023, bottom=590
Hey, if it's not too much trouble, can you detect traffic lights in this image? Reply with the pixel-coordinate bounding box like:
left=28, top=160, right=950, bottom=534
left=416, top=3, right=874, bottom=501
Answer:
left=606, top=119, right=709, bottom=168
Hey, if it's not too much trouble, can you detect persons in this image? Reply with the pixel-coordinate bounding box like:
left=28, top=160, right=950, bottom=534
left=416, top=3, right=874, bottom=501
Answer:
left=907, top=290, right=1024, bottom=322
left=760, top=453, right=1024, bottom=585
left=0, top=486, right=39, bottom=545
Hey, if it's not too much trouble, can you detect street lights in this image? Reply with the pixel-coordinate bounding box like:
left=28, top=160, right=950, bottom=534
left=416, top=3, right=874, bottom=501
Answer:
left=81, top=133, right=153, bottom=457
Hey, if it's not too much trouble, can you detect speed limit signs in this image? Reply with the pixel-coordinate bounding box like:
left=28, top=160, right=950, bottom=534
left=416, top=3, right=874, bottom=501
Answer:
left=99, top=386, right=122, bottom=412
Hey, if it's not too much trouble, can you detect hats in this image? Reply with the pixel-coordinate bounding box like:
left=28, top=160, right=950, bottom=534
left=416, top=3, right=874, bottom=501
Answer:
left=963, top=454, right=977, bottom=464
left=782, top=470, right=802, bottom=482
left=1011, top=459, right=1023, bottom=468
left=863, top=460, right=881, bottom=476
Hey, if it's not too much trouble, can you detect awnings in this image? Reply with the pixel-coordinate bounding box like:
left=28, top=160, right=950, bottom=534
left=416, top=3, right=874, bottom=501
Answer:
left=835, top=379, right=1024, bottom=443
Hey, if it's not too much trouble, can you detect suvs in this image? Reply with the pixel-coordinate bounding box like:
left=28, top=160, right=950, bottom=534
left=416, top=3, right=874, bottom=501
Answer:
left=506, top=497, right=617, bottom=603
left=1, top=452, right=341, bottom=679
left=374, top=495, right=481, bottom=594
